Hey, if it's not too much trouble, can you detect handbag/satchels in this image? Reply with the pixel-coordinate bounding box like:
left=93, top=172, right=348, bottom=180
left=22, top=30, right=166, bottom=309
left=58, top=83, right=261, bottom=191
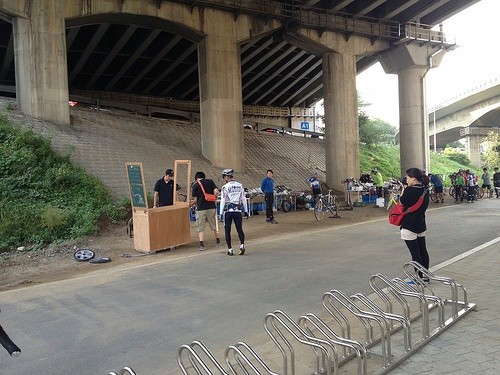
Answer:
left=389, top=202, right=404, bottom=226
left=205, top=193, right=216, bottom=201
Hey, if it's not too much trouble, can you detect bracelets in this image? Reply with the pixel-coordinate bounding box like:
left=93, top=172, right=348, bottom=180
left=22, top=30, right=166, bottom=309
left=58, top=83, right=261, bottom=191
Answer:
left=154, top=205, right=156, bottom=206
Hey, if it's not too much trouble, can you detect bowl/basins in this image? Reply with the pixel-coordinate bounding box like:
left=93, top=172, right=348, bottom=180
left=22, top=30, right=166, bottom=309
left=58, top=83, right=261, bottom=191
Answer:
left=354, top=202, right=366, bottom=206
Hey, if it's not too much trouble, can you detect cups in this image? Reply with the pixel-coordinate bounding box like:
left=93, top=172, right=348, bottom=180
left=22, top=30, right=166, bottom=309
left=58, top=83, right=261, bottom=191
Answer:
left=352, top=187, right=355, bottom=191
left=356, top=187, right=359, bottom=191
left=359, top=186, right=363, bottom=190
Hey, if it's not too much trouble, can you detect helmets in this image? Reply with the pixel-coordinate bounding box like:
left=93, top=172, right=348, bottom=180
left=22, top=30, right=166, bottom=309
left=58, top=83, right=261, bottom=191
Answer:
left=309, top=176, right=316, bottom=182
left=222, top=168, right=233, bottom=179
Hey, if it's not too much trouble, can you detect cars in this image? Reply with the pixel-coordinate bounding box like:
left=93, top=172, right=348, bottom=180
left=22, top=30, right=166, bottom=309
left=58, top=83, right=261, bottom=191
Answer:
left=260, top=128, right=280, bottom=134
left=142, top=111, right=189, bottom=122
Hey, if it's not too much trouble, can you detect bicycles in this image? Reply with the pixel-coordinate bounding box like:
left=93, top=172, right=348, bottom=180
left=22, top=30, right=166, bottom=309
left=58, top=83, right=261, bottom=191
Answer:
left=314, top=188, right=340, bottom=222
left=429, top=183, right=485, bottom=204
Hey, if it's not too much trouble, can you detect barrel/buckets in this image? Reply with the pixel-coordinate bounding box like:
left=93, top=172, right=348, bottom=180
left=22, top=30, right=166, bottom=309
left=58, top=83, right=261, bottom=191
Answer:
left=375, top=196, right=384, bottom=207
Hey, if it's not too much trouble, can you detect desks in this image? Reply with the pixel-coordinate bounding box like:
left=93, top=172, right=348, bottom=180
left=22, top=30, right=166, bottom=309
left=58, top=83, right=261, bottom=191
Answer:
left=346, top=190, right=371, bottom=207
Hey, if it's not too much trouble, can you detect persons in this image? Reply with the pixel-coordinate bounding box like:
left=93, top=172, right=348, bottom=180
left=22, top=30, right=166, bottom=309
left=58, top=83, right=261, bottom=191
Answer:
left=492, top=167, right=500, bottom=198
left=454, top=169, right=478, bottom=204
left=480, top=168, right=492, bottom=198
left=309, top=177, right=321, bottom=210
left=153, top=169, right=181, bottom=208
left=371, top=169, right=383, bottom=197
left=192, top=172, right=221, bottom=251
left=219, top=169, right=248, bottom=255
left=399, top=168, right=430, bottom=286
left=422, top=170, right=444, bottom=204
left=261, top=170, right=277, bottom=224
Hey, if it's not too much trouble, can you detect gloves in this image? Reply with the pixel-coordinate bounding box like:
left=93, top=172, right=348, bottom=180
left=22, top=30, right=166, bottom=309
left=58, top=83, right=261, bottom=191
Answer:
left=219, top=214, right=223, bottom=222
left=244, top=212, right=249, bottom=220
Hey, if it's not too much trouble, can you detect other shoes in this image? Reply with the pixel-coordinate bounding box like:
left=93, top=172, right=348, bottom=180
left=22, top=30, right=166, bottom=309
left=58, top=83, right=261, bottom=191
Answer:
left=200, top=244, right=207, bottom=250
left=315, top=208, right=321, bottom=212
left=239, top=244, right=245, bottom=255
left=406, top=278, right=431, bottom=288
left=267, top=216, right=278, bottom=224
left=434, top=200, right=440, bottom=203
left=226, top=248, right=233, bottom=255
left=441, top=199, right=444, bottom=203
left=216, top=241, right=222, bottom=247
